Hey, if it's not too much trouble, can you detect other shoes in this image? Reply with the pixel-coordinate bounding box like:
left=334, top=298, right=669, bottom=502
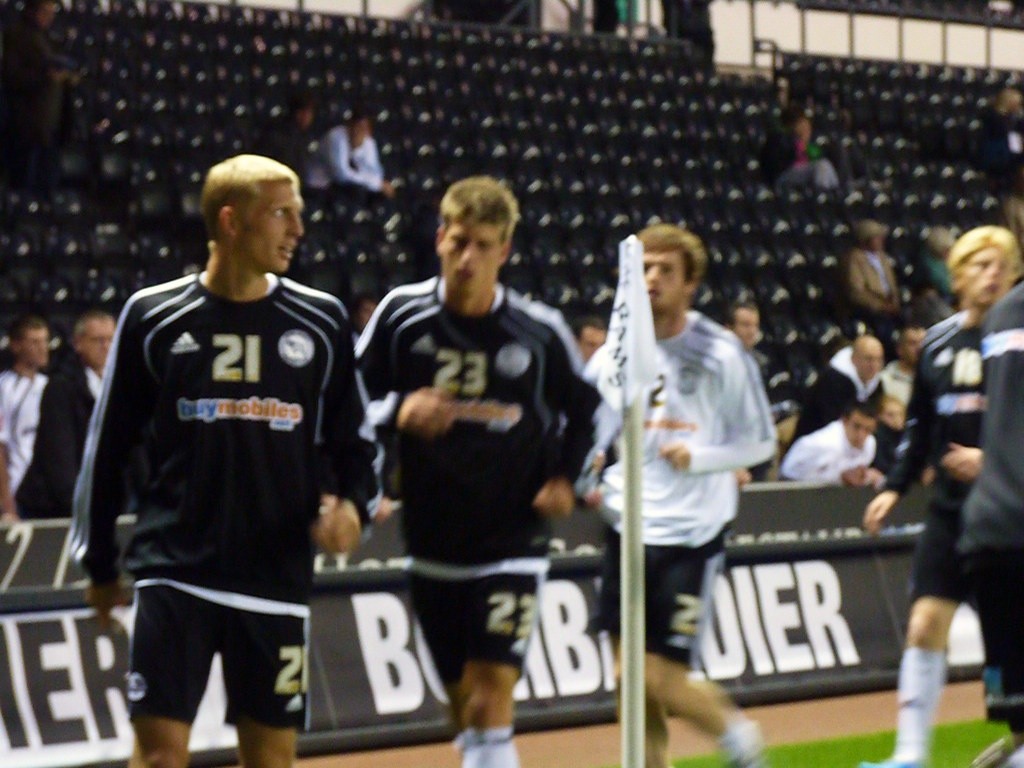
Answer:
left=859, top=760, right=922, bottom=768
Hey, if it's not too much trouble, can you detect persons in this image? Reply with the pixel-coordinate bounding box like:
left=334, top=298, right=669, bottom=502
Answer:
left=259, top=95, right=396, bottom=220
left=66, top=156, right=384, bottom=768
left=980, top=90, right=1024, bottom=259
left=313, top=178, right=1024, bottom=768
left=2, top=0, right=85, bottom=209
left=0, top=310, right=118, bottom=525
left=765, top=120, right=837, bottom=199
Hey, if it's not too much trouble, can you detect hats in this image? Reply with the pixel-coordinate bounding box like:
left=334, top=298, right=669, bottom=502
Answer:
left=855, top=219, right=886, bottom=242
left=946, top=222, right=1024, bottom=272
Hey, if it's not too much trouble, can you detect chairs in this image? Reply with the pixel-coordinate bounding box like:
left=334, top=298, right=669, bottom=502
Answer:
left=0, top=0, right=1024, bottom=407
